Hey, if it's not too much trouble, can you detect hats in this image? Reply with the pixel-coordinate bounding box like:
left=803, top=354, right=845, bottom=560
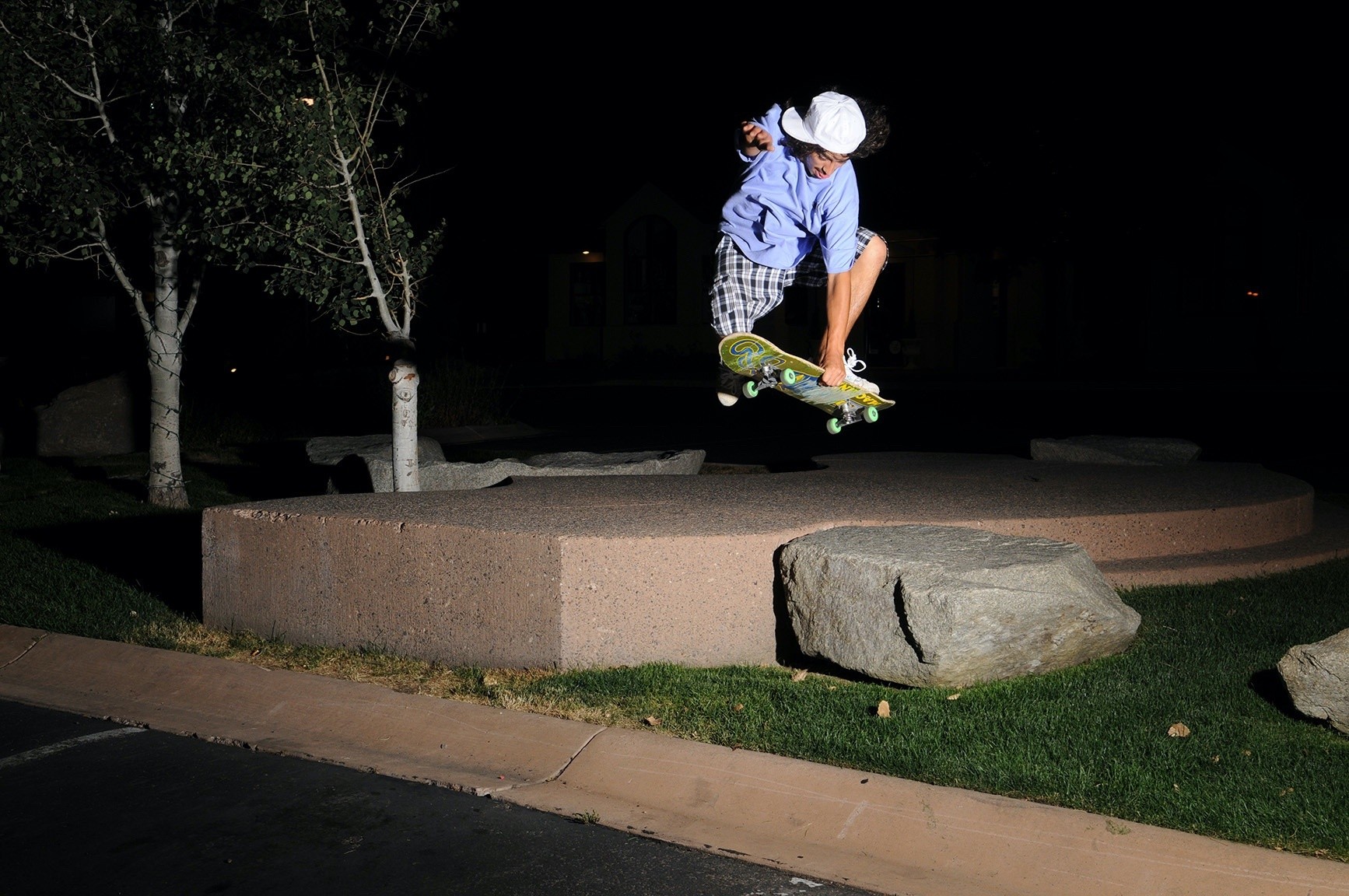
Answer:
left=781, top=91, right=867, bottom=154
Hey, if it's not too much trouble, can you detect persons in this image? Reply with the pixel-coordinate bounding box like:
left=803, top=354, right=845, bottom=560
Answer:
left=708, top=90, right=892, bottom=409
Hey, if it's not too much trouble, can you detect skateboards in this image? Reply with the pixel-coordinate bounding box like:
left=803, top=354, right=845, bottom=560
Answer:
left=719, top=333, right=895, bottom=434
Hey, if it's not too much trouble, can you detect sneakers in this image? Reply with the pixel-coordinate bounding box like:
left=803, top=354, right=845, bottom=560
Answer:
left=842, top=355, right=880, bottom=396
left=715, top=359, right=743, bottom=407
